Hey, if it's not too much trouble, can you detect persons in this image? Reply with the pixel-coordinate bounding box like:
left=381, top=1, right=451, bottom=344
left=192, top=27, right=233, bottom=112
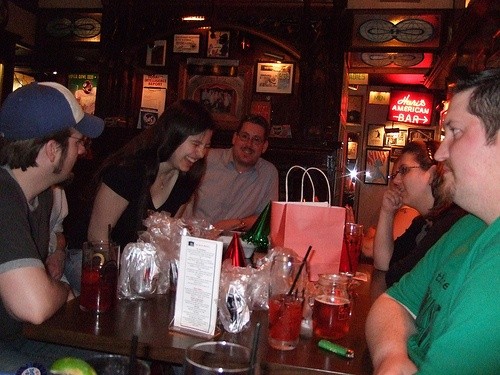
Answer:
left=373, top=138, right=467, bottom=287
left=181, top=111, right=279, bottom=235
left=0, top=83, right=121, bottom=375
left=65, top=100, right=215, bottom=294
left=366, top=68, right=500, bottom=375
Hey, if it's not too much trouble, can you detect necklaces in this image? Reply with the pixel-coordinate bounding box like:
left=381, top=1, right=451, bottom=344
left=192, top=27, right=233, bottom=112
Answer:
left=158, top=174, right=165, bottom=190
left=239, top=168, right=250, bottom=174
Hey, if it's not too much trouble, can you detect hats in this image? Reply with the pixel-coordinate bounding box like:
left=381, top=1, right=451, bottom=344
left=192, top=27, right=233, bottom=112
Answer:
left=0, top=81, right=104, bottom=140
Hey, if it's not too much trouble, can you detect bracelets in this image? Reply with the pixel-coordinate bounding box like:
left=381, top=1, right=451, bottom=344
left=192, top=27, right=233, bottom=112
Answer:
left=238, top=217, right=245, bottom=227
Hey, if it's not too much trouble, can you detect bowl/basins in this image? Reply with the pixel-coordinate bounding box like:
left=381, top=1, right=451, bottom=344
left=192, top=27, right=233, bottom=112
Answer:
left=217, top=235, right=259, bottom=259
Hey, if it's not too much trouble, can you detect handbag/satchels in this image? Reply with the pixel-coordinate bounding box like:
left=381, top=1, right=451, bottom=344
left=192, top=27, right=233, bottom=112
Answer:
left=270, top=166, right=346, bottom=279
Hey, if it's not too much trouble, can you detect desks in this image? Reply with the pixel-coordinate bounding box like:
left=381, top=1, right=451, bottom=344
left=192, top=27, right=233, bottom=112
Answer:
left=22, top=278, right=385, bottom=375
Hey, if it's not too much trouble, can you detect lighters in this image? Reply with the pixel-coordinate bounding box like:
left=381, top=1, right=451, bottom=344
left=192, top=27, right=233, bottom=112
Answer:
left=318, top=339, right=354, bottom=358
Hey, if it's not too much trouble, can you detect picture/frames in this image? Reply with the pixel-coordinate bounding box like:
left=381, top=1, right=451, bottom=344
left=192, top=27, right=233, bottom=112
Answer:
left=364, top=147, right=390, bottom=185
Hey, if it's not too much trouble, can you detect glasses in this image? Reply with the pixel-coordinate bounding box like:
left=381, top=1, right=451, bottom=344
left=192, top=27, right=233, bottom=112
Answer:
left=236, top=131, right=266, bottom=146
left=387, top=163, right=423, bottom=182
left=68, top=133, right=92, bottom=152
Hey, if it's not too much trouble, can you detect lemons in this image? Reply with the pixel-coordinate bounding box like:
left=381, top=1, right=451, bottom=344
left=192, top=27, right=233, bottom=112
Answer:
left=48, top=356, right=97, bottom=375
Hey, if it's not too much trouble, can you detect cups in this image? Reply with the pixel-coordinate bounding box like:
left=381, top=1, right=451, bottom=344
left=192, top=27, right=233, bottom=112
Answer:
left=86, top=354, right=151, bottom=375
left=268, top=255, right=308, bottom=351
left=183, top=341, right=262, bottom=375
left=345, top=223, right=364, bottom=271
left=313, top=273, right=352, bottom=341
left=79, top=240, right=120, bottom=313
